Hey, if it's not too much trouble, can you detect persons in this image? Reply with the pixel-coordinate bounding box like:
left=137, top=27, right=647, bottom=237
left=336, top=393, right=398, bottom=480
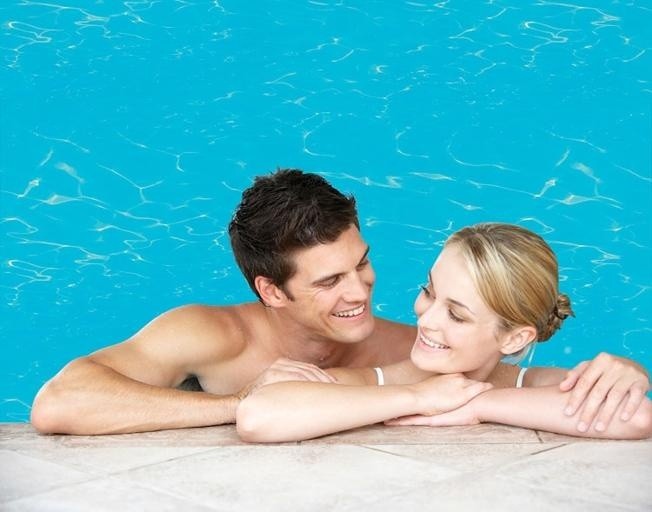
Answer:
left=235, top=221, right=652, bottom=445
left=28, top=169, right=651, bottom=438
